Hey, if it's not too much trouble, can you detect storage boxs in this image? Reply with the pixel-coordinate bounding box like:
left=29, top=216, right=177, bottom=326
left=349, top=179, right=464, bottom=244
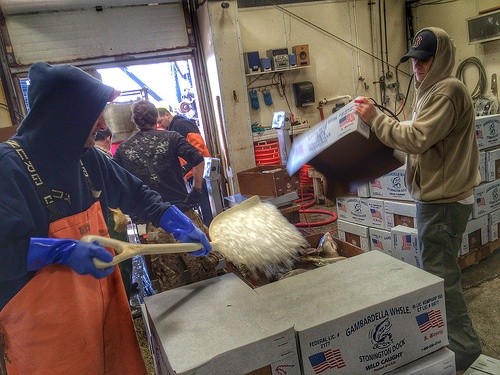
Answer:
left=139, top=97, right=500, bottom=375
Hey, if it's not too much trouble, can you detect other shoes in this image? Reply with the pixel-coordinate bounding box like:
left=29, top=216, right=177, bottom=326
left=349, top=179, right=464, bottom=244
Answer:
left=129, top=306, right=141, bottom=317
left=446, top=342, right=481, bottom=369
left=130, top=281, right=138, bottom=292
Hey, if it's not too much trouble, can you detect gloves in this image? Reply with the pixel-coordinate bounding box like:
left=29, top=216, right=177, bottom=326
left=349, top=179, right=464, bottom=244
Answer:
left=263, top=90, right=272, bottom=105
left=157, top=206, right=211, bottom=257
left=250, top=91, right=259, bottom=108
left=184, top=188, right=202, bottom=205
left=26, top=238, right=114, bottom=278
left=108, top=206, right=127, bottom=233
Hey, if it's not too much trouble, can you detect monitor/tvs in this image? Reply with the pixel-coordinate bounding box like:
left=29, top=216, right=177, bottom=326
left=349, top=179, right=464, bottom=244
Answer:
left=466, top=10, right=500, bottom=44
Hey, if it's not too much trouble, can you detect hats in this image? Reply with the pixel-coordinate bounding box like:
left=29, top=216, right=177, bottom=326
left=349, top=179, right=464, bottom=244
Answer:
left=399, top=30, right=437, bottom=62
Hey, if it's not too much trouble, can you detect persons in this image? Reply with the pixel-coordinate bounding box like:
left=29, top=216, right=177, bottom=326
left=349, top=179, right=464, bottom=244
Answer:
left=156, top=108, right=214, bottom=228
left=95, top=128, right=140, bottom=296
left=112, top=100, right=217, bottom=293
left=0, top=61, right=212, bottom=375
left=354, top=26, right=482, bottom=371
